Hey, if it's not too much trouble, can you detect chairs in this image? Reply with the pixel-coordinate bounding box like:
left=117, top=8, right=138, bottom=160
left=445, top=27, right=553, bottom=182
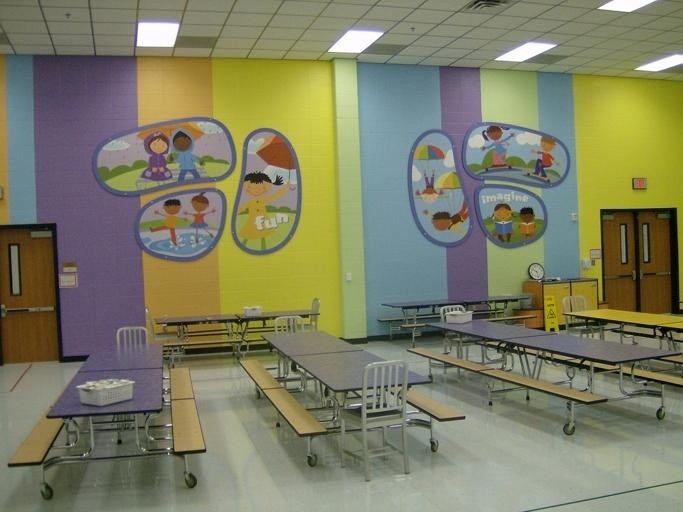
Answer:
left=117, top=326, right=148, bottom=351
left=274, top=316, right=307, bottom=380
left=338, top=360, right=410, bottom=481
left=146, top=307, right=180, bottom=359
left=304, top=298, right=320, bottom=330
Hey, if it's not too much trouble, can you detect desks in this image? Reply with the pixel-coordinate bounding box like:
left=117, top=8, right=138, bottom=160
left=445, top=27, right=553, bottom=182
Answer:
left=289, top=350, right=433, bottom=435
left=224, top=312, right=239, bottom=328
left=47, top=367, right=163, bottom=455
left=382, top=300, right=465, bottom=316
left=562, top=308, right=680, bottom=351
left=461, top=295, right=531, bottom=311
left=78, top=346, right=164, bottom=371
left=426, top=321, right=547, bottom=371
left=658, top=322, right=680, bottom=353
left=502, top=335, right=681, bottom=420
left=235, top=309, right=320, bottom=327
left=153, top=311, right=221, bottom=326
left=261, top=331, right=364, bottom=391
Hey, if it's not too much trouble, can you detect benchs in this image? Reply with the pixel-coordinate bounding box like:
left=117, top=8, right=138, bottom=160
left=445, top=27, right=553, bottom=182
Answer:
left=165, top=330, right=220, bottom=361
left=480, top=371, right=609, bottom=436
left=476, top=340, right=680, bottom=420
left=12, top=407, right=79, bottom=501
left=164, top=367, right=195, bottom=401
left=407, top=347, right=495, bottom=383
left=237, top=358, right=284, bottom=399
left=262, top=388, right=328, bottom=468
left=222, top=326, right=278, bottom=357
left=165, top=399, right=206, bottom=489
left=376, top=308, right=537, bottom=348
left=386, top=385, right=466, bottom=453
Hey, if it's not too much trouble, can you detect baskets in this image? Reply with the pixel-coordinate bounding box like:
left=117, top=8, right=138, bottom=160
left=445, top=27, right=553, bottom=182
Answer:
left=445, top=310, right=473, bottom=324
left=244, top=305, right=262, bottom=317
left=76, top=378, right=136, bottom=407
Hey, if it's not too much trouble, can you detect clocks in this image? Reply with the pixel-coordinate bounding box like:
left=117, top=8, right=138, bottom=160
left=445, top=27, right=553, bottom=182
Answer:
left=528, top=261, right=544, bottom=281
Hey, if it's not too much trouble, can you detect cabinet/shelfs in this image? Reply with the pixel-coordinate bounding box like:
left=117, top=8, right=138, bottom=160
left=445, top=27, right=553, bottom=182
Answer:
left=520, top=277, right=599, bottom=334
